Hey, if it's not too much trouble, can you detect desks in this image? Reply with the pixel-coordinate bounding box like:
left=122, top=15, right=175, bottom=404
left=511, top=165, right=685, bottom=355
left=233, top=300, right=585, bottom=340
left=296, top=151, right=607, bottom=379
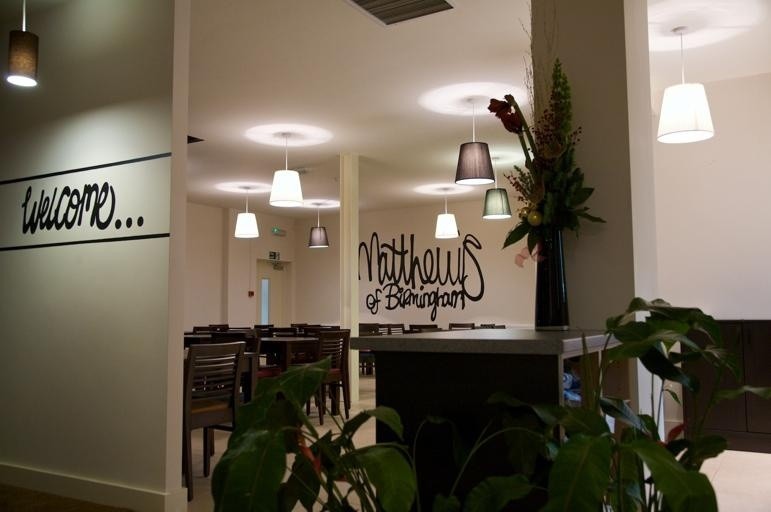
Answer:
left=350, top=326, right=622, bottom=512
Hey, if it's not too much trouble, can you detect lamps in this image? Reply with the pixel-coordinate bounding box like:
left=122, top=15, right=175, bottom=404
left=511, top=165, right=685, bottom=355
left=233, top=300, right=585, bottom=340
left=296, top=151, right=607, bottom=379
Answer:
left=434, top=188, right=458, bottom=238
left=455, top=99, right=496, bottom=185
left=234, top=187, right=260, bottom=238
left=6, top=0, right=39, bottom=87
left=309, top=202, right=328, bottom=248
left=657, top=26, right=715, bottom=144
left=483, top=157, right=513, bottom=220
left=268, top=132, right=304, bottom=208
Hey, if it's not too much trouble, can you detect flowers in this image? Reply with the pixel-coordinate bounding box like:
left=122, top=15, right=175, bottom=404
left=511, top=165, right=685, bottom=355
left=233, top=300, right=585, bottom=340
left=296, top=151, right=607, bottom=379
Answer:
left=488, top=0, right=606, bottom=268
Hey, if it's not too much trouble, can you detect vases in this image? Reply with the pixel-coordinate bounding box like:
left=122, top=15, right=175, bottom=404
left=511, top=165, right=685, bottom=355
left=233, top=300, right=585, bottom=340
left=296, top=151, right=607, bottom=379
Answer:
left=534, top=228, right=569, bottom=330
